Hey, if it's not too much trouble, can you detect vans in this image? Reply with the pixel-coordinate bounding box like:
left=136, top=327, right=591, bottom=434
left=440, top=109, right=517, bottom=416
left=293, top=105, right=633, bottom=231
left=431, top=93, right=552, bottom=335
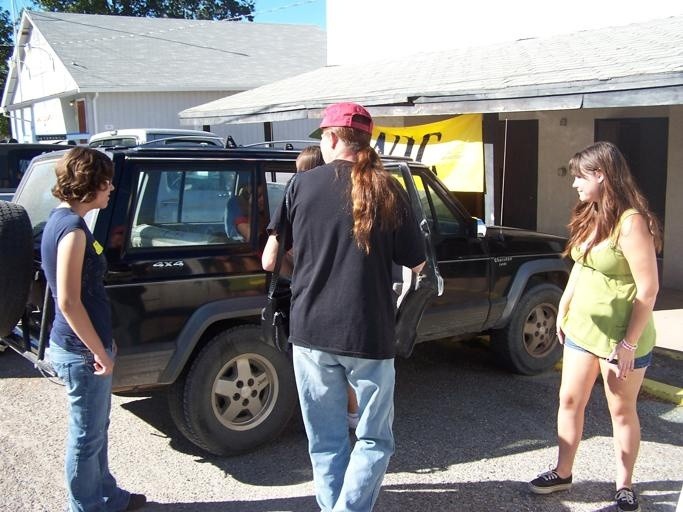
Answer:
left=87, top=128, right=226, bottom=148
left=0, top=143, right=85, bottom=203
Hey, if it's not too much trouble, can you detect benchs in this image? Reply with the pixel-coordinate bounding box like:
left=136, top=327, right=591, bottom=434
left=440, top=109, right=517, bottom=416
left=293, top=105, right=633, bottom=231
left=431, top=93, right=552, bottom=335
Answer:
left=130, top=223, right=237, bottom=248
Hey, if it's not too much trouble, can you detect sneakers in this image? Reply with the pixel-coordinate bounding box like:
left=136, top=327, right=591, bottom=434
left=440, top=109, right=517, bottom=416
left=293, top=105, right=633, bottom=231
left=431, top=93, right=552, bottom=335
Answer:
left=527, top=464, right=572, bottom=495
left=613, top=477, right=641, bottom=512
left=346, top=413, right=360, bottom=430
left=106, top=493, right=146, bottom=512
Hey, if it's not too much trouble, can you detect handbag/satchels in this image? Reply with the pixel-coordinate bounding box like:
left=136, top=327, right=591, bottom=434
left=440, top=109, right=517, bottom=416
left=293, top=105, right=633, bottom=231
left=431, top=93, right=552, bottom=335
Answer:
left=260, top=284, right=292, bottom=357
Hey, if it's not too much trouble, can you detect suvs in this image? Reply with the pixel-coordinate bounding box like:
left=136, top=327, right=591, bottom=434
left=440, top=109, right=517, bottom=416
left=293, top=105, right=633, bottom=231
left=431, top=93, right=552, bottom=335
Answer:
left=0, top=135, right=574, bottom=457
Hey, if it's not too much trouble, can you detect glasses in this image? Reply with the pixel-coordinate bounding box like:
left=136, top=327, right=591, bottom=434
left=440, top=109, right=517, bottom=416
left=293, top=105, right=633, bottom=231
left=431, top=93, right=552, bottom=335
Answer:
left=101, top=175, right=113, bottom=186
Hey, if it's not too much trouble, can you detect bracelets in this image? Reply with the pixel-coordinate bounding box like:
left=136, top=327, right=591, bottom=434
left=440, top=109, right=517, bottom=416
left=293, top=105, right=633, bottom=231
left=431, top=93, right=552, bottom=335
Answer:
left=621, top=338, right=638, bottom=352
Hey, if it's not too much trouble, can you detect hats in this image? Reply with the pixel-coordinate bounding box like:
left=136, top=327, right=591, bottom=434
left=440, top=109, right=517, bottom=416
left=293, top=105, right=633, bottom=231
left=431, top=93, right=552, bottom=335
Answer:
left=307, top=102, right=374, bottom=140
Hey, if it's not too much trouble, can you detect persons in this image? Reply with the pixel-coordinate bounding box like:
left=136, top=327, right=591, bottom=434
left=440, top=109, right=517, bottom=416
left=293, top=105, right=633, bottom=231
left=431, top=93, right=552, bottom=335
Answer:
left=528, top=141, right=658, bottom=512
left=235, top=179, right=267, bottom=244
left=40, top=146, right=146, bottom=512
left=262, top=101, right=427, bottom=512
left=295, top=145, right=361, bottom=429
